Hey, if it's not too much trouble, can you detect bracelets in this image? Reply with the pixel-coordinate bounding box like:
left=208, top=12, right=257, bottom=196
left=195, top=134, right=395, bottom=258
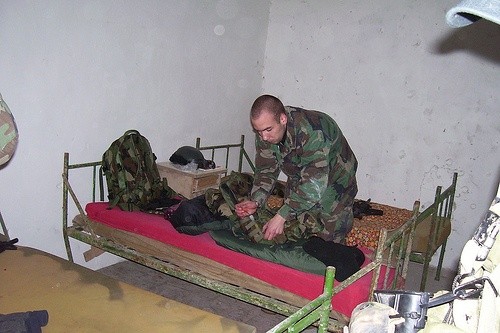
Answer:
left=255, top=201, right=261, bottom=208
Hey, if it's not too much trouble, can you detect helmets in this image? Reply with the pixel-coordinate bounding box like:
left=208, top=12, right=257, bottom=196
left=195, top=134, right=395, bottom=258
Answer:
left=169, top=145, right=205, bottom=168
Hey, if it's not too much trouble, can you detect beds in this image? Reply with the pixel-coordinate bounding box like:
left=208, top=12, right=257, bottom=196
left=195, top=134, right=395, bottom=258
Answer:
left=0, top=209, right=337, bottom=333
left=62, top=150, right=421, bottom=333
left=195, top=135, right=459, bottom=291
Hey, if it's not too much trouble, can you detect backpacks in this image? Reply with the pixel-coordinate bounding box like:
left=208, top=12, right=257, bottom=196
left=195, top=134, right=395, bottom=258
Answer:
left=101, top=129, right=176, bottom=210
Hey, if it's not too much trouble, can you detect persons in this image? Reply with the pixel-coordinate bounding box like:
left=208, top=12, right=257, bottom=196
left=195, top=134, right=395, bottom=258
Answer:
left=233, top=94, right=358, bottom=244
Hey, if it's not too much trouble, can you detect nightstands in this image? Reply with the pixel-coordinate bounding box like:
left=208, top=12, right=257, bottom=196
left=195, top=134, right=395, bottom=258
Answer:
left=156, top=160, right=221, bottom=199
